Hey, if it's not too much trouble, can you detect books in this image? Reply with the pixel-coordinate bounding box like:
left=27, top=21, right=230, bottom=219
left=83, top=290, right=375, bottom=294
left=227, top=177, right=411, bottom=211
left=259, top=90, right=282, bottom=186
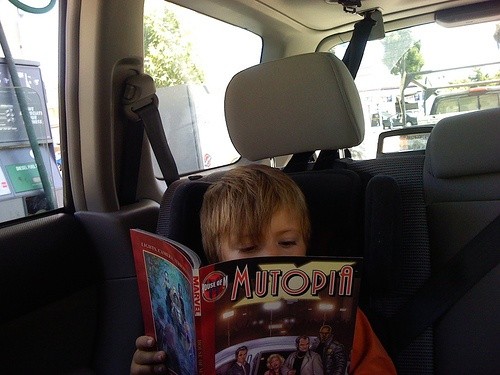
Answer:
left=130, top=228, right=364, bottom=375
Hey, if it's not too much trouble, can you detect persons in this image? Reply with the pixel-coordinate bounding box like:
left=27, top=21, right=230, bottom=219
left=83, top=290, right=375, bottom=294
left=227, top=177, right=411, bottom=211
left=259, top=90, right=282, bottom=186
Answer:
left=129, top=165, right=397, bottom=375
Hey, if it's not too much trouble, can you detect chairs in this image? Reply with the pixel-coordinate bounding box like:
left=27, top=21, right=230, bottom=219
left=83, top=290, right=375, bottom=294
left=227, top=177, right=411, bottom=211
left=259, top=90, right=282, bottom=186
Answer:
left=157, top=52, right=500, bottom=375
